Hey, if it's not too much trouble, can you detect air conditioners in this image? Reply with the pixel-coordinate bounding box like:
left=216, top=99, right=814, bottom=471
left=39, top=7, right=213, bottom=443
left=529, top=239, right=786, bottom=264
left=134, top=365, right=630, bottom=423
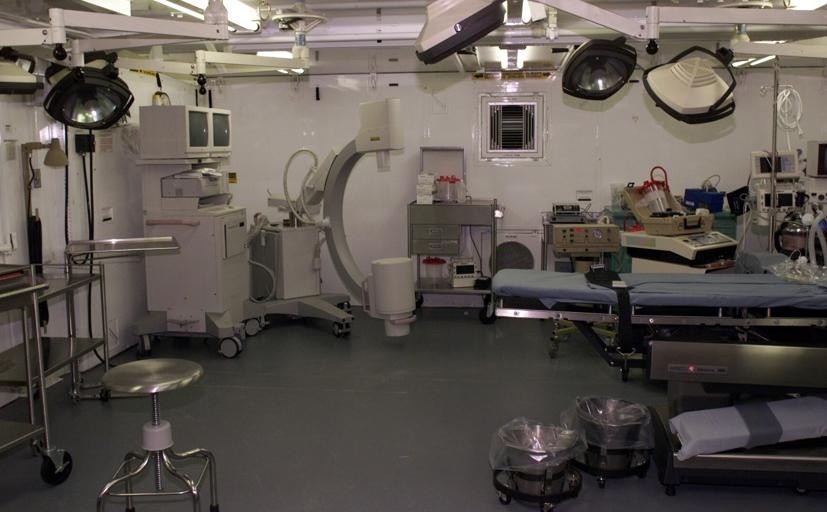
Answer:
left=481, top=232, right=544, bottom=278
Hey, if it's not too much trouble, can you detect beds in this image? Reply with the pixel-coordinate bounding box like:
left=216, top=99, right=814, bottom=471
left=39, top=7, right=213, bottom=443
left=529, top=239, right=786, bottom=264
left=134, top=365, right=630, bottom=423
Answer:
left=492, top=268, right=827, bottom=381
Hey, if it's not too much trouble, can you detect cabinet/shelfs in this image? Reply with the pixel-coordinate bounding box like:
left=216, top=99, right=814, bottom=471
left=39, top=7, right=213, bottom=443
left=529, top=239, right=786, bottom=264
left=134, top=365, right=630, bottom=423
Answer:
left=408, top=199, right=498, bottom=326
left=0, top=264, right=73, bottom=486
left=0, top=262, right=110, bottom=455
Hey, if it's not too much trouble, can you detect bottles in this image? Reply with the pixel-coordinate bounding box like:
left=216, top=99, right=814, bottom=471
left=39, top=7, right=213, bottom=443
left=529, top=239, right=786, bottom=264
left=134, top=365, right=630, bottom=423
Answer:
left=435, top=175, right=465, bottom=202
left=422, top=258, right=446, bottom=287
left=639, top=181, right=669, bottom=216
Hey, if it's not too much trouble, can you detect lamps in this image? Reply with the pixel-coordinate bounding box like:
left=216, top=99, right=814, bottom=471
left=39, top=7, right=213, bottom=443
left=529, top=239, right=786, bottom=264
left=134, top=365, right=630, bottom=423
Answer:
left=642, top=39, right=659, bottom=55
left=643, top=48, right=737, bottom=122
left=519, top=1, right=549, bottom=26
left=21, top=139, right=68, bottom=167
left=416, top=1, right=506, bottom=66
left=0, top=48, right=43, bottom=96
left=44, top=52, right=135, bottom=130
left=562, top=34, right=639, bottom=99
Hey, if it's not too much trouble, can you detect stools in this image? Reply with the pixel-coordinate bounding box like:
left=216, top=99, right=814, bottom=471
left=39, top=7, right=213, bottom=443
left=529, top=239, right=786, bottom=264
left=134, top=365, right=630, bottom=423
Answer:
left=96, top=359, right=218, bottom=512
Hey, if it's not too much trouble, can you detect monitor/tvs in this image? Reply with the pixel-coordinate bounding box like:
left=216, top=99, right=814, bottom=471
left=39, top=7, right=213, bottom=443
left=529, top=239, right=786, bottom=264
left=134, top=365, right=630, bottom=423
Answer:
left=138, top=105, right=210, bottom=159
left=210, top=107, right=232, bottom=158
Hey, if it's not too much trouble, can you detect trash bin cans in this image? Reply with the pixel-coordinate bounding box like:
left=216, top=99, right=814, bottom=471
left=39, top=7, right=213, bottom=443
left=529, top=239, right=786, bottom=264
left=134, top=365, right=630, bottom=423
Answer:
left=570, top=394, right=656, bottom=489
left=494, top=419, right=582, bottom=512
